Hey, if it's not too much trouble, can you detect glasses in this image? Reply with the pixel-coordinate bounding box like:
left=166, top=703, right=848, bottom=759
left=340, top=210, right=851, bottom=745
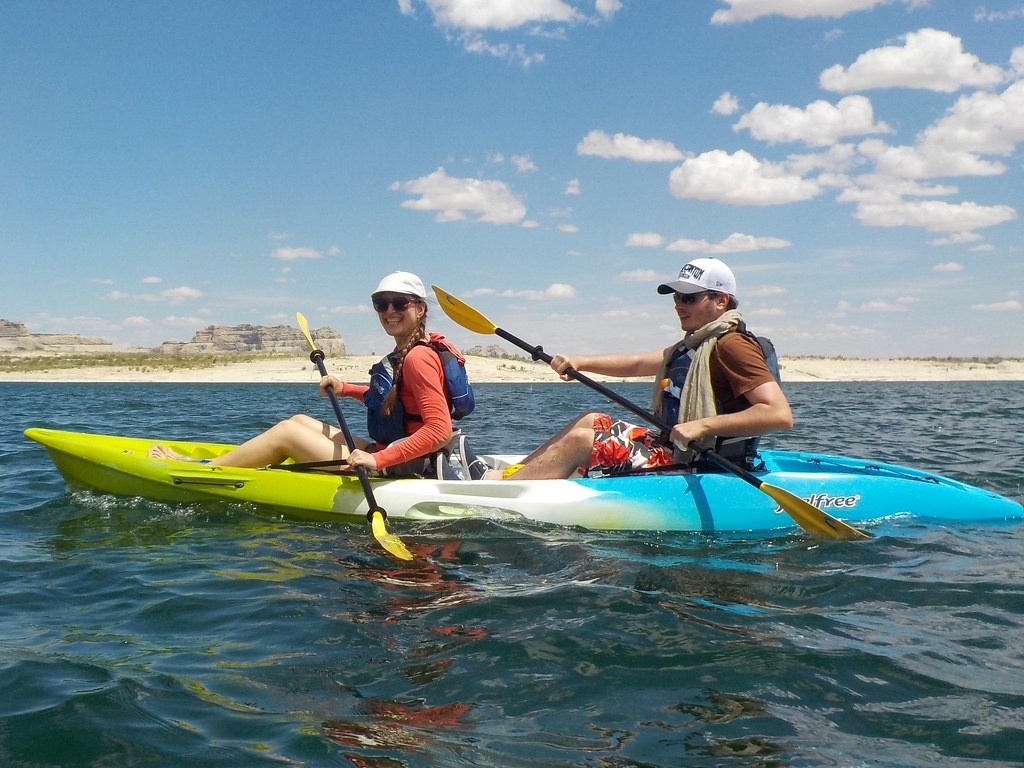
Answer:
left=372, top=297, right=419, bottom=312
left=673, top=291, right=722, bottom=305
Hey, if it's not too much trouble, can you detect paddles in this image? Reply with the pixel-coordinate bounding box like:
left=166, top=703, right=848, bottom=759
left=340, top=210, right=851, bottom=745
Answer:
left=297, top=312, right=414, bottom=561
left=431, top=283, right=870, bottom=541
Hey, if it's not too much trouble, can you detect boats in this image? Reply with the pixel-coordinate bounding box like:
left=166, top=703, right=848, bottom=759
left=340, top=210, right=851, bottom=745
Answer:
left=22, top=424, right=1024, bottom=535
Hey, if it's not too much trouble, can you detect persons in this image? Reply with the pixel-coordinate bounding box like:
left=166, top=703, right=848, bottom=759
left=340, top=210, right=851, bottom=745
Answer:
left=437, top=257, right=793, bottom=481
left=147, top=269, right=453, bottom=479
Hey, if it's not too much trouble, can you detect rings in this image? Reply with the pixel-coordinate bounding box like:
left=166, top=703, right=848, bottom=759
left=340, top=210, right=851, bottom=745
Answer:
left=352, top=458, right=355, bottom=462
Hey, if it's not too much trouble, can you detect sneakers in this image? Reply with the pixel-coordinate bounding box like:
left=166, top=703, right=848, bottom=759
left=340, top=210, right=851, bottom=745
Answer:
left=437, top=452, right=462, bottom=480
left=460, top=435, right=492, bottom=480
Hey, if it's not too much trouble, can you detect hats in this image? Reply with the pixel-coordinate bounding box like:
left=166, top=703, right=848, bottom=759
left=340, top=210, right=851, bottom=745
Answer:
left=657, top=258, right=736, bottom=297
left=371, top=271, right=426, bottom=298
